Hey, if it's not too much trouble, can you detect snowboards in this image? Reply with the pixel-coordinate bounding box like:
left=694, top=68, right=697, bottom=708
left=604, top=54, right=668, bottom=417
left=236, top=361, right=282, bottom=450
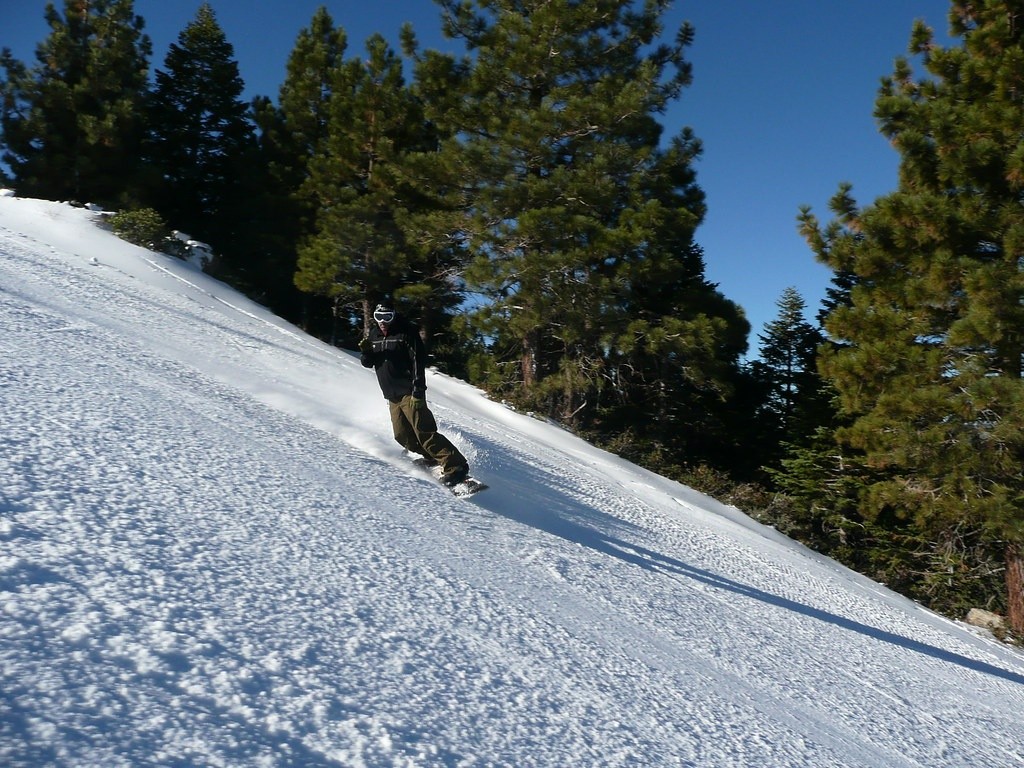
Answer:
left=416, top=457, right=489, bottom=496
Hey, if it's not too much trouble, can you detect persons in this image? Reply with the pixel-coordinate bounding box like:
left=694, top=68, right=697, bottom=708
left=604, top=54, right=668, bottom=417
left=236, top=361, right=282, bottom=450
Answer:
left=358, top=299, right=469, bottom=484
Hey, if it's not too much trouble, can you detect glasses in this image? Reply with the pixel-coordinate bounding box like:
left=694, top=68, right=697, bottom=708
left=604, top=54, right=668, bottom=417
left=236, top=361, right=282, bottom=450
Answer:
left=374, top=310, right=395, bottom=323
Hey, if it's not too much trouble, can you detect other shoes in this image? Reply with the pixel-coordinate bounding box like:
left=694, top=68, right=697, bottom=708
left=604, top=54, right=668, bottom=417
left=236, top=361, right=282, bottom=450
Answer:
left=444, top=463, right=469, bottom=485
left=414, top=458, right=438, bottom=467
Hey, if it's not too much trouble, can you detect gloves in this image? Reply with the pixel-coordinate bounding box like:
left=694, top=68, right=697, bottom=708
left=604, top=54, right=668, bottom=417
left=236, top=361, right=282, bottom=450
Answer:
left=358, top=337, right=373, bottom=353
left=409, top=390, right=426, bottom=412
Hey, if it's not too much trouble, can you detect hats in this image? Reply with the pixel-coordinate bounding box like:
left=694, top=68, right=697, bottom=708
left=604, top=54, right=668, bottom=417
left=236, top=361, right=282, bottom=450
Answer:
left=375, top=300, right=394, bottom=313
